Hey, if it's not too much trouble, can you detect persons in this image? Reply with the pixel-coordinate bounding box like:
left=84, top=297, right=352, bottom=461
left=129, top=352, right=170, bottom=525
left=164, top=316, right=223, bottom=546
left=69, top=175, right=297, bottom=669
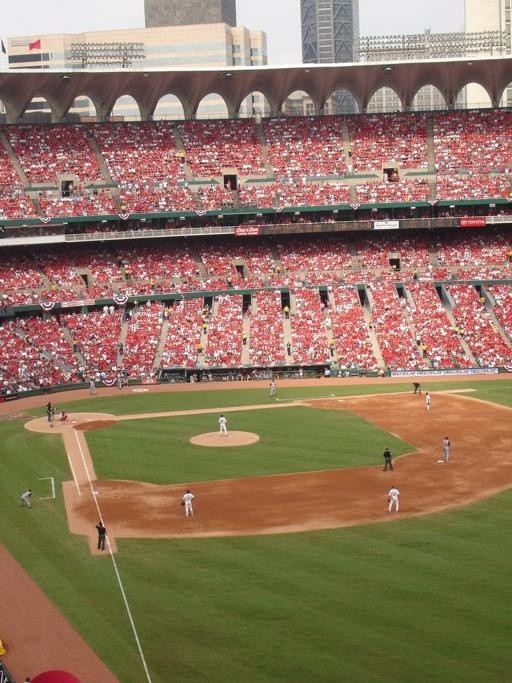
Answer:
left=19, top=488, right=32, bottom=507
left=46, top=401, right=53, bottom=421
left=382, top=447, right=394, bottom=471
left=387, top=485, right=401, bottom=512
left=424, top=391, right=431, bottom=410
left=0, top=107, right=512, bottom=399
left=181, top=488, right=195, bottom=516
left=24, top=676, right=31, bottom=683
left=58, top=409, right=68, bottom=421
left=217, top=414, right=228, bottom=435
left=442, top=436, right=452, bottom=461
left=95, top=521, right=108, bottom=550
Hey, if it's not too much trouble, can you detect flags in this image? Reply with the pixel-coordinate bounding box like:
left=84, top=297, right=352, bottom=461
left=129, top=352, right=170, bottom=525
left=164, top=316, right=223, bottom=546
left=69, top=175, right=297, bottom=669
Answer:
left=1, top=39, right=6, bottom=54
left=28, top=39, right=41, bottom=51
